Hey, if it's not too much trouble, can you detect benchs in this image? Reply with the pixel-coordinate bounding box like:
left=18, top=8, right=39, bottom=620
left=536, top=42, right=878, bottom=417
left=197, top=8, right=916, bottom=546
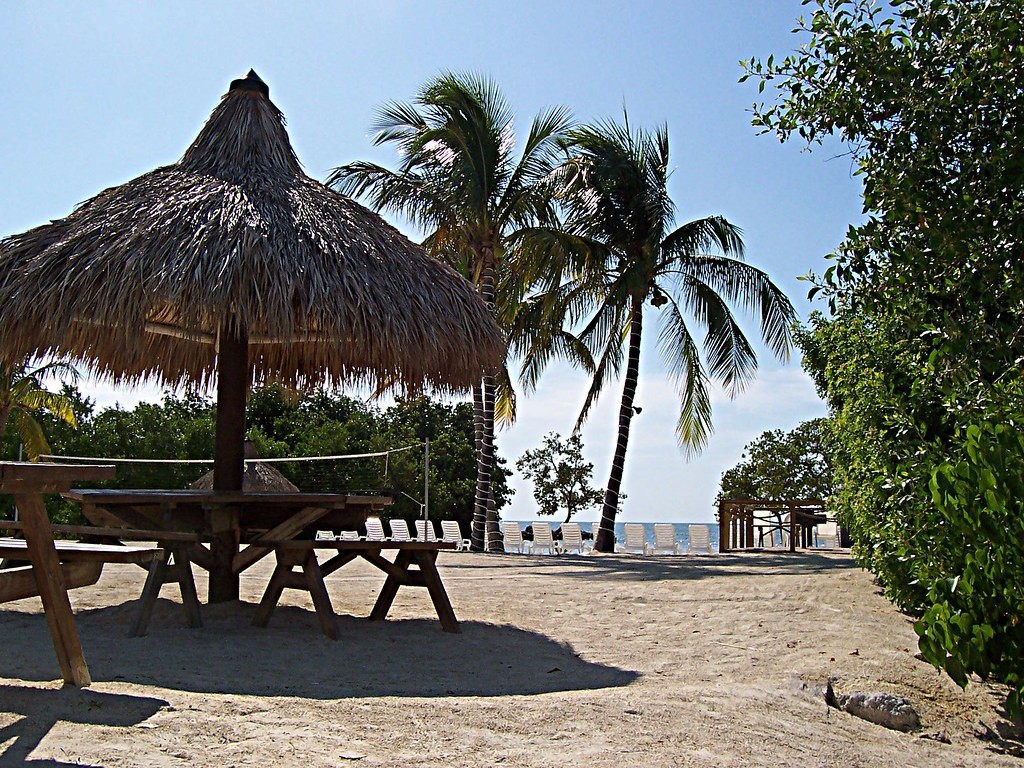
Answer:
left=250, top=538, right=462, bottom=641
left=0, top=520, right=216, bottom=638
left=0, top=538, right=165, bottom=590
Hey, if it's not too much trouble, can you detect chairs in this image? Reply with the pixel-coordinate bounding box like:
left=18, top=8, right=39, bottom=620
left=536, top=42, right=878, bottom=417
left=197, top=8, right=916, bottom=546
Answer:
left=315, top=530, right=340, bottom=541
left=687, top=524, right=714, bottom=556
left=530, top=522, right=560, bottom=555
left=619, top=524, right=650, bottom=556
left=340, top=531, right=365, bottom=541
left=365, top=519, right=391, bottom=542
left=415, top=520, right=442, bottom=542
left=813, top=506, right=841, bottom=548
left=591, top=522, right=618, bottom=552
left=389, top=519, right=417, bottom=542
left=471, top=521, right=489, bottom=552
left=651, top=524, right=682, bottom=555
left=441, top=520, right=471, bottom=551
left=502, top=521, right=530, bottom=553
left=753, top=510, right=775, bottom=547
left=560, top=523, right=589, bottom=554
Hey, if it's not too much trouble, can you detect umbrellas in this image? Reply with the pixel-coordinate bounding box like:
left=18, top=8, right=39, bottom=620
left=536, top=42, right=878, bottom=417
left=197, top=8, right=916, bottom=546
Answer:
left=0, top=68, right=511, bottom=602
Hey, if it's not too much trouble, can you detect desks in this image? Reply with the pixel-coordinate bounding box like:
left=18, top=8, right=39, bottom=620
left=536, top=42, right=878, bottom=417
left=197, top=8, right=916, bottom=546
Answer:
left=0, top=460, right=116, bottom=688
left=60, top=489, right=393, bottom=574
